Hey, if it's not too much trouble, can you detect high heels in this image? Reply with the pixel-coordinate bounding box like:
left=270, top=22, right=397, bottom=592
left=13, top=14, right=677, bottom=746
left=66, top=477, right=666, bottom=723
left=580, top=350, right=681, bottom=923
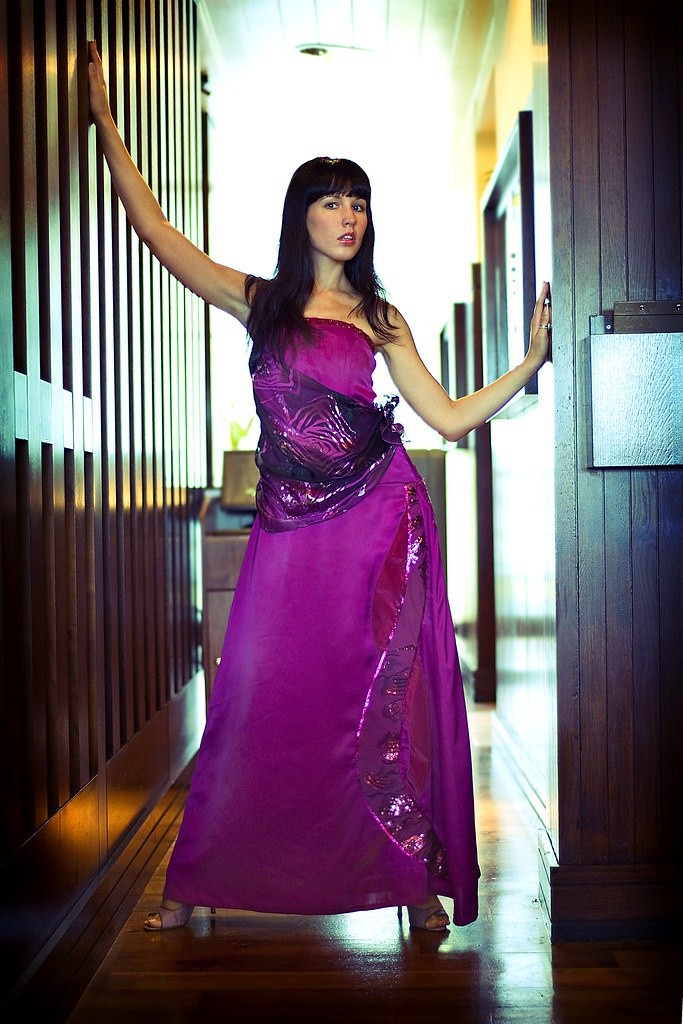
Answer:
left=397, top=902, right=449, bottom=933
left=144, top=903, right=216, bottom=931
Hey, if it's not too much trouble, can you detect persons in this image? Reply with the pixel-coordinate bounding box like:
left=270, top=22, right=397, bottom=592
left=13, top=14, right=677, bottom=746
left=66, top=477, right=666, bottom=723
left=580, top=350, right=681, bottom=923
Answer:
left=87, top=38, right=553, bottom=933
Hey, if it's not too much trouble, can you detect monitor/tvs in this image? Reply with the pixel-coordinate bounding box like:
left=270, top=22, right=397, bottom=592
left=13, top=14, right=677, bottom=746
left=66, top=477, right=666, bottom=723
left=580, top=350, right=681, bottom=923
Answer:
left=220, top=451, right=261, bottom=528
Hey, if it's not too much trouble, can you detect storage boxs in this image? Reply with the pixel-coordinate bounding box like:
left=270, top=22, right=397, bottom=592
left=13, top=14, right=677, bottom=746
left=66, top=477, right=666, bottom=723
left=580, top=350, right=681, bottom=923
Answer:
left=612, top=300, right=683, bottom=333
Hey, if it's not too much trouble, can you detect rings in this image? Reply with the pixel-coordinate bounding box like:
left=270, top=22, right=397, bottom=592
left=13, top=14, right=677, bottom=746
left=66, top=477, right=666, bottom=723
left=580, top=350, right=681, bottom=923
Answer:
left=540, top=325, right=548, bottom=328
